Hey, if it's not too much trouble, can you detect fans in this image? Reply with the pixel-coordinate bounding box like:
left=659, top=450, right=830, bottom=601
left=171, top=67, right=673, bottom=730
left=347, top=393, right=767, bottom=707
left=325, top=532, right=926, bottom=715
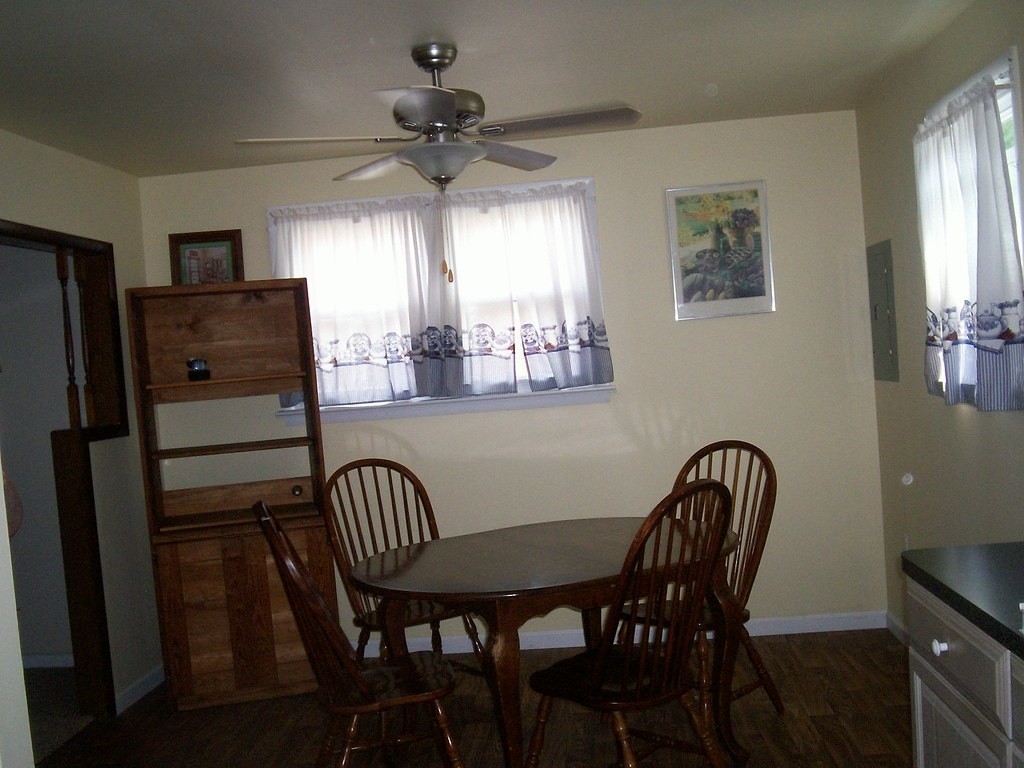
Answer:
left=234, top=44, right=643, bottom=182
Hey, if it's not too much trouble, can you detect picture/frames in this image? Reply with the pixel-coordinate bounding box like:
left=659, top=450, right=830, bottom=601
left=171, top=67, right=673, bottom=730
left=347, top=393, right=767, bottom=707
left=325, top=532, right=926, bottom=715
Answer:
left=665, top=179, right=776, bottom=321
left=167, top=229, right=244, bottom=286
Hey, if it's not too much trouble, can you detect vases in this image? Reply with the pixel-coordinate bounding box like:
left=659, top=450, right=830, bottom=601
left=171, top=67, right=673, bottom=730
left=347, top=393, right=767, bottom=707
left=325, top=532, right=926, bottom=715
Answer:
left=727, top=229, right=743, bottom=247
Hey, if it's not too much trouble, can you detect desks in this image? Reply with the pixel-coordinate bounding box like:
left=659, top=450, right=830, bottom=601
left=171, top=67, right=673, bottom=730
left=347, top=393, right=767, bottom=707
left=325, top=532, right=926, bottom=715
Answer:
left=349, top=517, right=740, bottom=768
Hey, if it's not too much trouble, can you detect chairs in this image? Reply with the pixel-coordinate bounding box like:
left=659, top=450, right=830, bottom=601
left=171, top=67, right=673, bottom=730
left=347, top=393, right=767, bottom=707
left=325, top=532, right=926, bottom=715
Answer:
left=253, top=458, right=488, bottom=768
left=528, top=441, right=784, bottom=768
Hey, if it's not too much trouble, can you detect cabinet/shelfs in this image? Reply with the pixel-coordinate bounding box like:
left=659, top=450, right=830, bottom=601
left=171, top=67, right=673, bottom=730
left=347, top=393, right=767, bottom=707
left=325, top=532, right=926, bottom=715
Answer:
left=900, top=542, right=1024, bottom=768
left=124, top=277, right=340, bottom=712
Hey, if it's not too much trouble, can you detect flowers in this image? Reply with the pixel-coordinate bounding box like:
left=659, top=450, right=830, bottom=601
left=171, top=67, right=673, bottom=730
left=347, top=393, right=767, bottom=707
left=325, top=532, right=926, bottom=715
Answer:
left=723, top=207, right=760, bottom=231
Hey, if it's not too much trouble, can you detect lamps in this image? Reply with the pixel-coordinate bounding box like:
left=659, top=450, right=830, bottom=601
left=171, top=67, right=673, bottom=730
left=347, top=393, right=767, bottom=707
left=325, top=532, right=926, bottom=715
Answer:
left=394, top=143, right=488, bottom=192
